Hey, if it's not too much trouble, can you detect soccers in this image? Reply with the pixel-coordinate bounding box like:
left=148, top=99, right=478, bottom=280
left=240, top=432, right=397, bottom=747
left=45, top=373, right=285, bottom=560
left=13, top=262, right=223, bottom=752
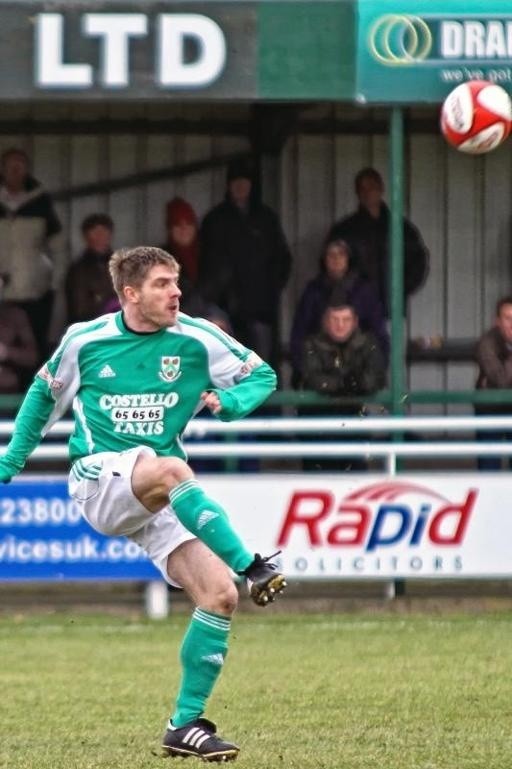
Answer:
left=438, top=79, right=511, bottom=153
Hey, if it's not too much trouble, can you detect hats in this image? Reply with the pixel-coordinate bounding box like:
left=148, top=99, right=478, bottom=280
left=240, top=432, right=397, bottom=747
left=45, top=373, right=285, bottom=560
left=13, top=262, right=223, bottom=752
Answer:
left=166, top=197, right=198, bottom=227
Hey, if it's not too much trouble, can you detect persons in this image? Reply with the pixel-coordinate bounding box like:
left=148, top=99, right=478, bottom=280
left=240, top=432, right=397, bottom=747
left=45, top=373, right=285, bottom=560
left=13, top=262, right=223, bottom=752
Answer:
left=1, top=144, right=65, bottom=346
left=1, top=244, right=287, bottom=764
left=154, top=194, right=207, bottom=324
left=469, top=297, right=512, bottom=472
left=170, top=303, right=264, bottom=473
left=286, top=235, right=394, bottom=372
left=62, top=210, right=121, bottom=323
left=288, top=299, right=391, bottom=469
left=191, top=150, right=295, bottom=370
left=2, top=271, right=41, bottom=394
left=323, top=168, right=431, bottom=299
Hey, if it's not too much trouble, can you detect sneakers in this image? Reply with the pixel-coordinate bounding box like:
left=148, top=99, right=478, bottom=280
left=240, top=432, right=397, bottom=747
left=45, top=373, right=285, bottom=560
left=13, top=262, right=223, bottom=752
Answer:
left=244, top=554, right=288, bottom=607
left=162, top=717, right=239, bottom=760
